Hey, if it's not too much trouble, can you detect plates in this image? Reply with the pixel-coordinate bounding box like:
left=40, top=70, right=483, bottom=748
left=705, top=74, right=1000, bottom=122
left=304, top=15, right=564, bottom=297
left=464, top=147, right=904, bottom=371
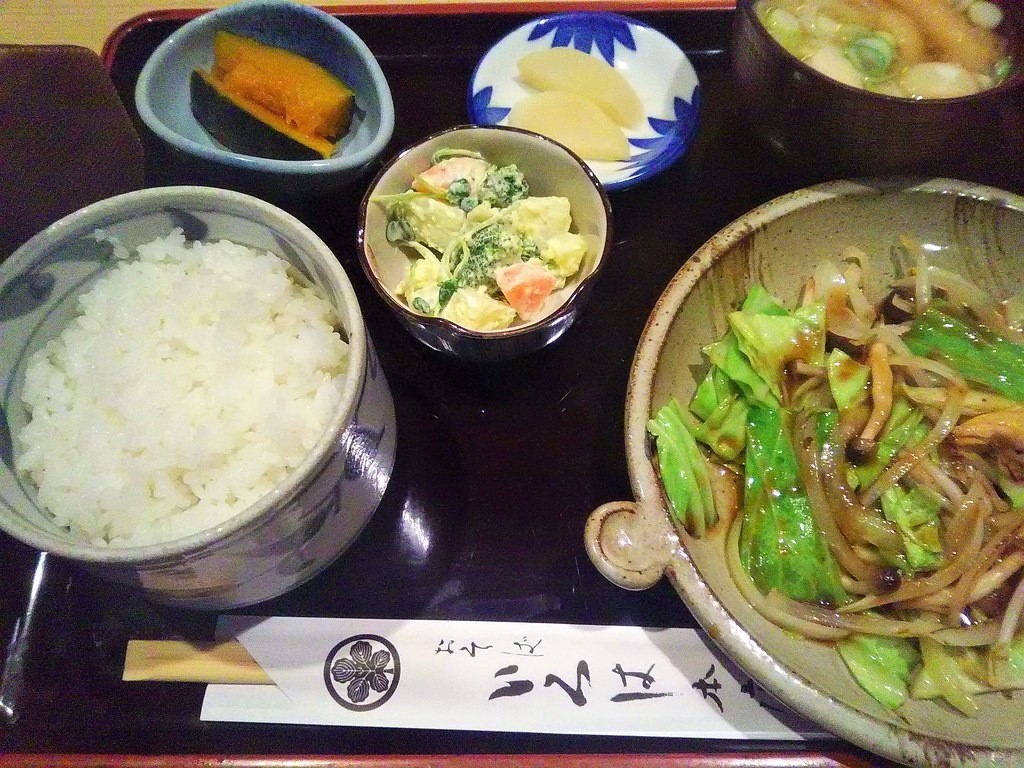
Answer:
left=137, top=5, right=392, bottom=175
left=586, top=174, right=1023, bottom=768
left=467, top=10, right=702, bottom=192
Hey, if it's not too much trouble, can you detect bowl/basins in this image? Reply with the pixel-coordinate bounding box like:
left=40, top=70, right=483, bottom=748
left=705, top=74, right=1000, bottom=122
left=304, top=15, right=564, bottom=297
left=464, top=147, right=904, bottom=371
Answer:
left=1, top=185, right=399, bottom=612
left=360, top=125, right=616, bottom=368
left=731, top=3, right=1018, bottom=191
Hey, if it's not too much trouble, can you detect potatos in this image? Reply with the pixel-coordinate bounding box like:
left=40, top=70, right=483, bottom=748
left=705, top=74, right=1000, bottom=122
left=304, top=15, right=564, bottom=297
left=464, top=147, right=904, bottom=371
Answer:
left=374, top=152, right=585, bottom=335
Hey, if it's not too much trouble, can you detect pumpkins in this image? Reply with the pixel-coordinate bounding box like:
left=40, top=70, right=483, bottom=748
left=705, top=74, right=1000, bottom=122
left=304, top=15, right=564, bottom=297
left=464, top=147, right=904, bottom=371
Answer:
left=192, top=30, right=358, bottom=163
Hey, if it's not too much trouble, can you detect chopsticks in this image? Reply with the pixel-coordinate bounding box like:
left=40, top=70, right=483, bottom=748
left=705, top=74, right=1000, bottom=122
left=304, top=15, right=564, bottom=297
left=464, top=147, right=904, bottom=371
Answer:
left=117, top=637, right=284, bottom=689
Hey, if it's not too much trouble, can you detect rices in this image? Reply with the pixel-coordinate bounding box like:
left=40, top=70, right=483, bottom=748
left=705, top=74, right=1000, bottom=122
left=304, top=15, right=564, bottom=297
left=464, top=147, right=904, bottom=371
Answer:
left=13, top=219, right=348, bottom=551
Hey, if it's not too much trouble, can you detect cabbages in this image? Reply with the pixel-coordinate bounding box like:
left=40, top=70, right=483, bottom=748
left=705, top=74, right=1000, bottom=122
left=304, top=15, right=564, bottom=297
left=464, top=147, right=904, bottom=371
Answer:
left=650, top=231, right=1024, bottom=722
